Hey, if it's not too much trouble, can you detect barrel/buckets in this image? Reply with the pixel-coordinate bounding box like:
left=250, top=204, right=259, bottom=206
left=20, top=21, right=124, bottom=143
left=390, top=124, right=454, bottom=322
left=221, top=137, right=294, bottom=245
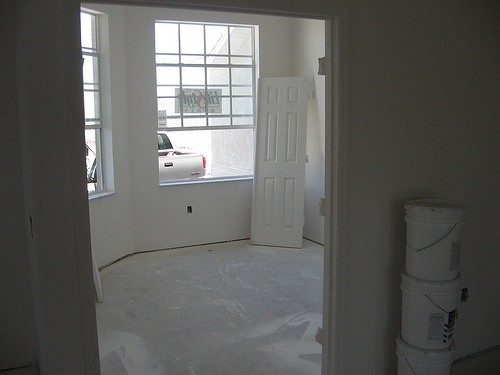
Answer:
left=402, top=198, right=468, bottom=282
left=394, top=336, right=455, bottom=375
left=399, top=272, right=462, bottom=350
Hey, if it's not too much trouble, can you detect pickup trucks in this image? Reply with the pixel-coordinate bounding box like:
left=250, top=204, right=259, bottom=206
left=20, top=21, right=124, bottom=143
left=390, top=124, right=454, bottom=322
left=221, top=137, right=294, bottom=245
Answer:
left=156, top=131, right=207, bottom=182
